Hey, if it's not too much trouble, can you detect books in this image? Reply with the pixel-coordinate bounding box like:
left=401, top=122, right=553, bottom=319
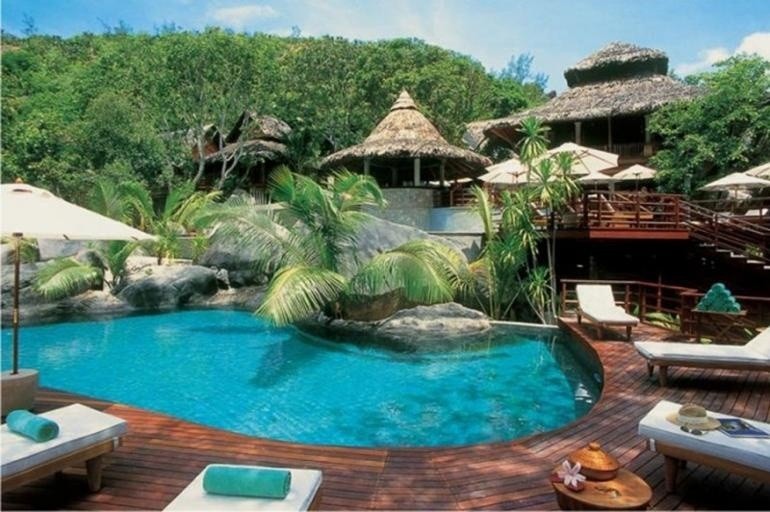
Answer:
left=716, top=418, right=768, bottom=438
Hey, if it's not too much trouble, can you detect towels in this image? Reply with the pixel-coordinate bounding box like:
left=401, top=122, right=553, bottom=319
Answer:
left=695, top=282, right=743, bottom=313
left=5, top=409, right=59, bottom=442
left=202, top=465, right=291, bottom=500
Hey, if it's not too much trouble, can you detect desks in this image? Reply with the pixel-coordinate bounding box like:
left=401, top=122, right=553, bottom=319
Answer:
left=549, top=460, right=654, bottom=510
left=691, top=308, right=748, bottom=344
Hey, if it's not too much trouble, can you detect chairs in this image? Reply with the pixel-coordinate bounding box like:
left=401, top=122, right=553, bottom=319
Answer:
left=637, top=398, right=770, bottom=494
left=575, top=284, right=640, bottom=342
left=159, top=462, right=323, bottom=512
left=0, top=402, right=128, bottom=494
left=634, top=325, right=770, bottom=387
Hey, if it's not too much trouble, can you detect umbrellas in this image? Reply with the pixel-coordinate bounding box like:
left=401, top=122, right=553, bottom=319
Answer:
left=0, top=177, right=161, bottom=378
left=476, top=140, right=658, bottom=197
left=698, top=171, right=770, bottom=215
left=745, top=161, right=770, bottom=181
